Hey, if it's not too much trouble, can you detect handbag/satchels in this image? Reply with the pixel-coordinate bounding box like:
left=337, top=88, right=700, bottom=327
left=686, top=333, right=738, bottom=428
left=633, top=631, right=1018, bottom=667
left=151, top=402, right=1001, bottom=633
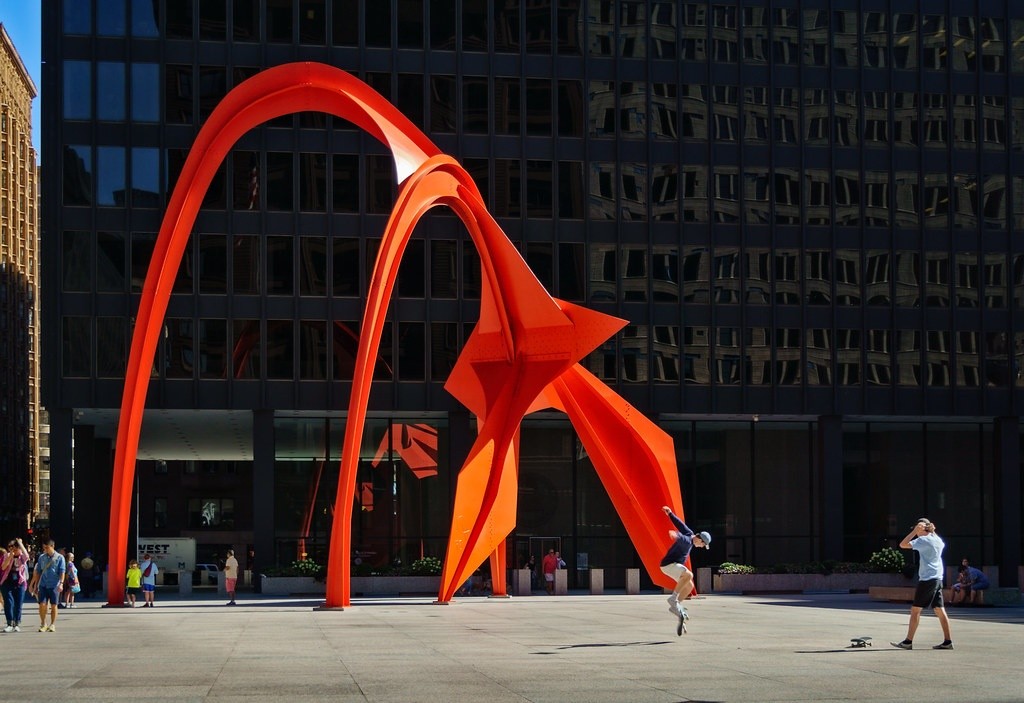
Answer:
left=143, top=562, right=152, bottom=577
left=69, top=584, right=81, bottom=593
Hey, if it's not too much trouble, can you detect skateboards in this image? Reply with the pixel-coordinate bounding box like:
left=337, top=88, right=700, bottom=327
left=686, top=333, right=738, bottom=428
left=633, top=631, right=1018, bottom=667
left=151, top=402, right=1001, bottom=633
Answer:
left=676, top=609, right=688, bottom=636
left=850, top=636, right=873, bottom=648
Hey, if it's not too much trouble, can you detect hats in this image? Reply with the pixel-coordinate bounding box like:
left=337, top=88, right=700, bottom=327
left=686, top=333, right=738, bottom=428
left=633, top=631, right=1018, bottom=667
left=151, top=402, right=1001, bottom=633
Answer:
left=911, top=518, right=930, bottom=530
left=700, top=532, right=712, bottom=549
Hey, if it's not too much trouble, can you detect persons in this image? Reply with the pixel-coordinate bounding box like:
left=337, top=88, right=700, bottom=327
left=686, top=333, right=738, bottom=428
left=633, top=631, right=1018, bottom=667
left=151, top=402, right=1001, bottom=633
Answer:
left=524, top=555, right=537, bottom=594
left=57, top=549, right=81, bottom=608
left=29, top=563, right=40, bottom=601
left=542, top=549, right=559, bottom=594
left=0, top=538, right=29, bottom=631
left=660, top=506, right=711, bottom=615
left=484, top=582, right=491, bottom=595
left=945, top=556, right=989, bottom=606
left=126, top=560, right=142, bottom=607
left=555, top=552, right=566, bottom=569
left=81, top=552, right=96, bottom=598
left=890, top=518, right=954, bottom=650
left=34, top=539, right=66, bottom=631
left=223, top=550, right=238, bottom=605
left=455, top=578, right=472, bottom=597
left=140, top=554, right=159, bottom=607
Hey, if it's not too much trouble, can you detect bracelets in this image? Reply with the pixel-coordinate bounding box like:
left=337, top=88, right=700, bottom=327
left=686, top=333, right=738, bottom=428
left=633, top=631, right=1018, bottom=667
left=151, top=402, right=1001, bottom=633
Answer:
left=60, top=582, right=63, bottom=584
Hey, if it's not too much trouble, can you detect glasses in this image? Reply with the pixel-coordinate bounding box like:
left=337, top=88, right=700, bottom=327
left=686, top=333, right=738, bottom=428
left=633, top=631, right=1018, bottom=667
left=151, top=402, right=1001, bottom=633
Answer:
left=551, top=552, right=554, bottom=553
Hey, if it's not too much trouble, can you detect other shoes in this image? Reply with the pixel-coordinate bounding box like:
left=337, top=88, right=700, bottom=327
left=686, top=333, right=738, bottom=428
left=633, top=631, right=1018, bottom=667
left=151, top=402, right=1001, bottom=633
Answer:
left=58, top=603, right=64, bottom=608
left=668, top=598, right=679, bottom=612
left=3, top=626, right=12, bottom=632
left=669, top=607, right=681, bottom=618
left=143, top=603, right=148, bottom=607
left=65, top=605, right=77, bottom=609
left=890, top=640, right=912, bottom=650
left=14, top=626, right=20, bottom=632
left=150, top=603, right=153, bottom=607
left=48, top=624, right=55, bottom=632
left=226, top=601, right=236, bottom=606
left=932, top=641, right=953, bottom=649
left=39, top=626, right=48, bottom=632
